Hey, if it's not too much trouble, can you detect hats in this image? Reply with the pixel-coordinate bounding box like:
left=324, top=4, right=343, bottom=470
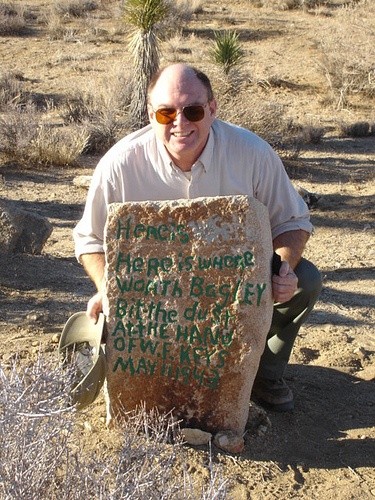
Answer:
left=57, top=309, right=108, bottom=409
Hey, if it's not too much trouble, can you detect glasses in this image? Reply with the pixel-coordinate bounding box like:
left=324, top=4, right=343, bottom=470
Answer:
left=149, top=104, right=208, bottom=124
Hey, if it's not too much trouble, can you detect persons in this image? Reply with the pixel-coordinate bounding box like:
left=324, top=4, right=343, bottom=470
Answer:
left=73, top=64, right=322, bottom=404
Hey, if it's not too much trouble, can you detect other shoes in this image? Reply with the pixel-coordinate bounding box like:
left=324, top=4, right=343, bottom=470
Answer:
left=251, top=376, right=294, bottom=412
